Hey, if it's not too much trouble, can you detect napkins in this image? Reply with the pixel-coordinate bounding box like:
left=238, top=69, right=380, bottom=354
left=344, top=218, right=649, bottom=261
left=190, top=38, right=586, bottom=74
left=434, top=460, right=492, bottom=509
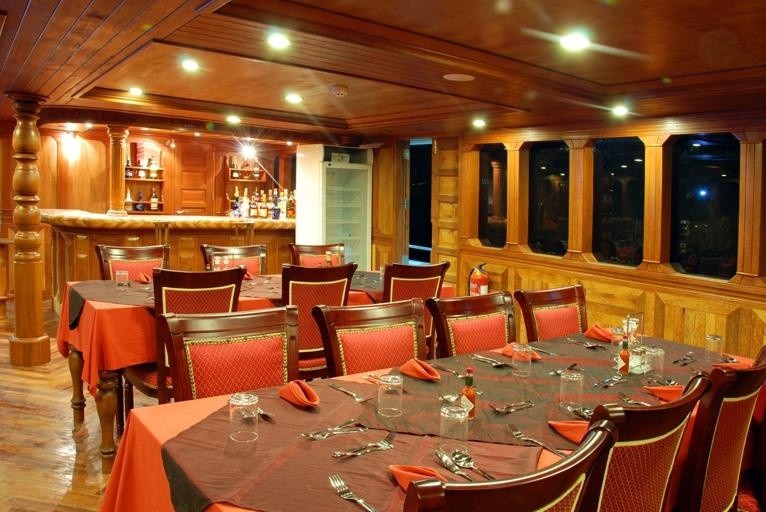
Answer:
left=501, top=342, right=542, bottom=363
left=547, top=420, right=590, bottom=445
left=388, top=462, right=442, bottom=492
left=710, top=360, right=750, bottom=371
left=584, top=324, right=617, bottom=344
left=276, top=379, right=321, bottom=409
left=643, top=382, right=685, bottom=403
left=399, top=361, right=441, bottom=383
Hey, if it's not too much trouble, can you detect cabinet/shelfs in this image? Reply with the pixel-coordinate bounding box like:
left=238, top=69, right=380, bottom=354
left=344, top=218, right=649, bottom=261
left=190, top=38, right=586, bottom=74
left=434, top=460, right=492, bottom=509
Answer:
left=125, top=165, right=163, bottom=214
left=227, top=155, right=267, bottom=203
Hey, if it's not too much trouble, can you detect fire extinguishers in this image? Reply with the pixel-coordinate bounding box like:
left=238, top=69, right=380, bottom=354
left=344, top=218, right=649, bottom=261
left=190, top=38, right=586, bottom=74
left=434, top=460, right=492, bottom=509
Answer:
left=468, top=262, right=489, bottom=296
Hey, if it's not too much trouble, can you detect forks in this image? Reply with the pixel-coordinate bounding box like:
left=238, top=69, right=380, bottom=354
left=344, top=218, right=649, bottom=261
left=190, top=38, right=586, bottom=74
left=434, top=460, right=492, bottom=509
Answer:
left=329, top=382, right=373, bottom=404
left=506, top=422, right=570, bottom=460
left=302, top=418, right=367, bottom=439
left=256, top=406, right=275, bottom=422
left=488, top=400, right=535, bottom=414
left=434, top=449, right=478, bottom=488
left=323, top=472, right=378, bottom=511
left=470, top=353, right=515, bottom=369
left=617, top=392, right=651, bottom=408
left=674, top=352, right=697, bottom=367
left=430, top=363, right=472, bottom=379
left=564, top=337, right=605, bottom=351
left=331, top=432, right=396, bottom=462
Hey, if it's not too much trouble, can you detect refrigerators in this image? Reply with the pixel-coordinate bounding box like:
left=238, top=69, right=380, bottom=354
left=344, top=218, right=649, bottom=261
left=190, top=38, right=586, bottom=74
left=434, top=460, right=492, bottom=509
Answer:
left=294, top=143, right=374, bottom=276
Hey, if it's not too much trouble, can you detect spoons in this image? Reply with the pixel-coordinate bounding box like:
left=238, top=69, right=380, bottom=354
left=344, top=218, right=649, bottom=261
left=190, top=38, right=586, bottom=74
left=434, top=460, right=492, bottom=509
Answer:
left=235, top=408, right=256, bottom=423
left=452, top=449, right=500, bottom=482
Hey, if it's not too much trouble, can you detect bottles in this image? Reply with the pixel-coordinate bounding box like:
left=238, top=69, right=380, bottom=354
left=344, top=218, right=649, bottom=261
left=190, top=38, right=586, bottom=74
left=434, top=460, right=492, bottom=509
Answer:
left=461, top=367, right=476, bottom=420
left=124, top=157, right=164, bottom=212
left=228, top=156, right=295, bottom=219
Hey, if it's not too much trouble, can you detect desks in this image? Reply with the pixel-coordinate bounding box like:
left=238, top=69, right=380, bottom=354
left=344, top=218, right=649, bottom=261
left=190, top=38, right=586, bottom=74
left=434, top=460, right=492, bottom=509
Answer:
left=99, top=330, right=765, bottom=511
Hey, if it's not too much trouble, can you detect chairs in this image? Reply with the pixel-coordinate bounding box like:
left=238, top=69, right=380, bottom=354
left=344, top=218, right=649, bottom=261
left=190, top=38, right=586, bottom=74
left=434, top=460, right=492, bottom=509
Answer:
left=200, top=243, right=266, bottom=275
left=125, top=264, right=246, bottom=434
left=427, top=290, right=514, bottom=358
left=514, top=284, right=589, bottom=343
left=281, top=261, right=357, bottom=378
left=401, top=419, right=618, bottom=512
left=384, top=261, right=450, bottom=360
left=679, top=346, right=766, bottom=511
left=161, top=304, right=299, bottom=404
left=576, top=368, right=712, bottom=512
left=96, top=242, right=169, bottom=281
left=288, top=243, right=345, bottom=268
left=313, top=296, right=428, bottom=378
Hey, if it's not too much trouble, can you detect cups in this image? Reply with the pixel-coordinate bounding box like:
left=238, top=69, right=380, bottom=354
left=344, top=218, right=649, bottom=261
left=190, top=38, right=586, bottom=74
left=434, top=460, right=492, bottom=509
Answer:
left=113, top=248, right=387, bottom=304
left=559, top=370, right=584, bottom=412
left=511, top=344, right=533, bottom=378
left=377, top=375, right=404, bottom=418
left=226, top=392, right=263, bottom=444
left=440, top=404, right=471, bottom=453
left=704, top=336, right=722, bottom=365
left=611, top=316, right=671, bottom=384
